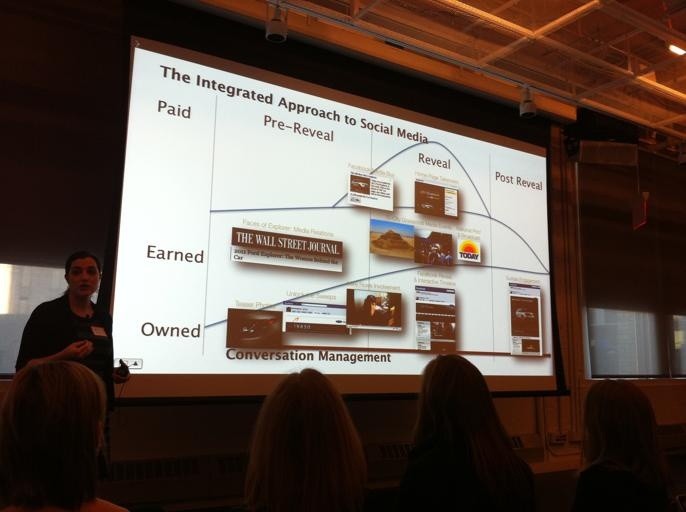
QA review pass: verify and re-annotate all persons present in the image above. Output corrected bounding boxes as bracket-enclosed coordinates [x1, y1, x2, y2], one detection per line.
[571, 379, 676, 510]
[1, 357, 129, 512]
[14, 249, 131, 475]
[244, 368, 376, 511]
[389, 354, 540, 510]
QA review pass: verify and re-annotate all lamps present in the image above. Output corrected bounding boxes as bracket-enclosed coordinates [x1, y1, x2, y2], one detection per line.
[517, 82, 537, 122]
[264, 0, 292, 49]
